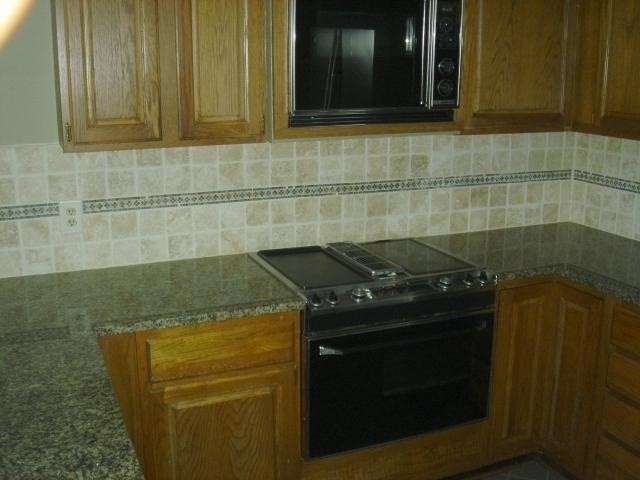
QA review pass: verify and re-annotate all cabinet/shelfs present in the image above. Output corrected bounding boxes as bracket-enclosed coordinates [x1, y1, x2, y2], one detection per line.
[490, 274, 640, 480]
[51, 0, 270, 154]
[97, 309, 303, 480]
[460, 0, 640, 142]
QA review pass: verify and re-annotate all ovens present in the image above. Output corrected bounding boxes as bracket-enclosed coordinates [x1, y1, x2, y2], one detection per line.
[305, 291, 494, 461]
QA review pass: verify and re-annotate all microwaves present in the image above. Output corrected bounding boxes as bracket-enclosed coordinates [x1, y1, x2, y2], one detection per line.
[291, 0, 461, 122]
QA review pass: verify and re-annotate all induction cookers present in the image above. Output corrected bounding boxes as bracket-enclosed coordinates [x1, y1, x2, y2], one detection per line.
[252, 236, 501, 310]
